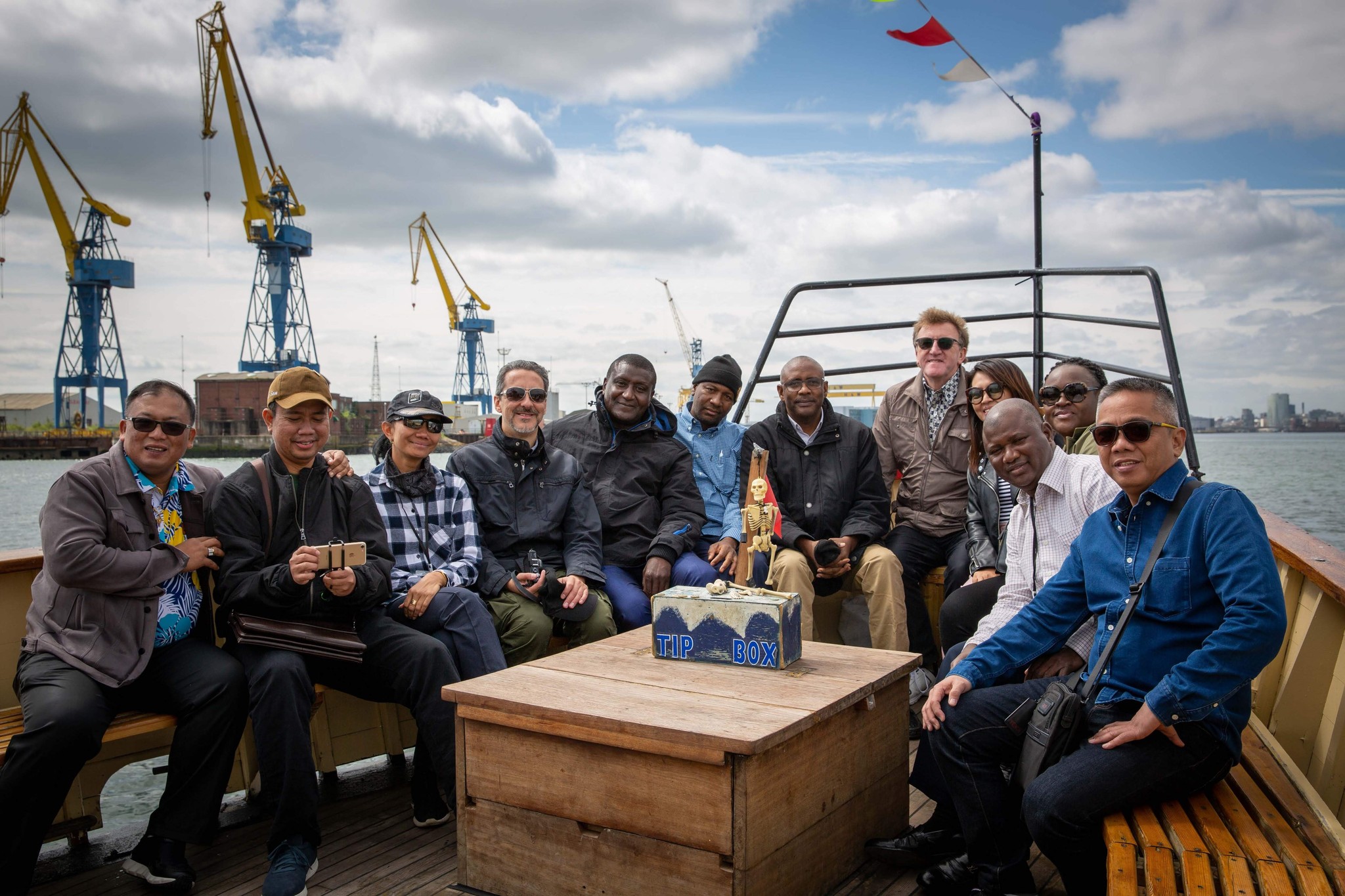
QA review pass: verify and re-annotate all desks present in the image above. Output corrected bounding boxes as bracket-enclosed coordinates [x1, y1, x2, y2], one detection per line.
[441, 624, 921, 896]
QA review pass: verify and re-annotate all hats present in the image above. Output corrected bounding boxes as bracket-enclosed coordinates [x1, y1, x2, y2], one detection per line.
[810, 540, 845, 596]
[387, 390, 453, 425]
[541, 567, 598, 621]
[267, 365, 335, 413]
[692, 353, 743, 403]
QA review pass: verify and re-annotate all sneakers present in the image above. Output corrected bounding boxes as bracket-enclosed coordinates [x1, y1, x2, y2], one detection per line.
[121, 834, 197, 893]
[260, 834, 320, 896]
[908, 667, 936, 705]
[407, 773, 451, 827]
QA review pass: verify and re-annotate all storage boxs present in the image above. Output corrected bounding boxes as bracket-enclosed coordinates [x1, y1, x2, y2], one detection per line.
[651, 583, 803, 669]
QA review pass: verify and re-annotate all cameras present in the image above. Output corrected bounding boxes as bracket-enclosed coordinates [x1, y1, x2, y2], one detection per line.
[524, 549, 543, 586]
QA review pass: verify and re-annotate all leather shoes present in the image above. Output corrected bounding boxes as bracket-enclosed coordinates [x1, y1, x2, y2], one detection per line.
[916, 851, 980, 896]
[863, 823, 967, 857]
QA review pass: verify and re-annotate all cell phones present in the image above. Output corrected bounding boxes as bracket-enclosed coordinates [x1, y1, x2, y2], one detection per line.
[314, 542, 367, 569]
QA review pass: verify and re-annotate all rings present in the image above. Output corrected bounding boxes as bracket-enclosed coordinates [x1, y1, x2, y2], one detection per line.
[208, 546, 214, 556]
[329, 585, 333, 589]
[411, 599, 417, 605]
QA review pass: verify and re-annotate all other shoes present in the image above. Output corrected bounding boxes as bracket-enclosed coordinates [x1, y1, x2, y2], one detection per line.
[909, 706, 922, 738]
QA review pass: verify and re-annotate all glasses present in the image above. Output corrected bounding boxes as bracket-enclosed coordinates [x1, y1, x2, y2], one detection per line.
[1089, 420, 1177, 448]
[1038, 382, 1099, 406]
[390, 415, 443, 434]
[499, 386, 547, 403]
[915, 337, 960, 351]
[783, 377, 826, 392]
[126, 417, 193, 436]
[963, 381, 1005, 405]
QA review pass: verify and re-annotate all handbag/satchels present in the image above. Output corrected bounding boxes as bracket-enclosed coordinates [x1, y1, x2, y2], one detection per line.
[1016, 676, 1082, 794]
[229, 607, 367, 662]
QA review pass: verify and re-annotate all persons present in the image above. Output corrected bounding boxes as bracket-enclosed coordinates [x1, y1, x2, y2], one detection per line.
[960, 359, 1066, 588]
[869, 311, 972, 706]
[939, 357, 1108, 656]
[368, 389, 507, 816]
[670, 354, 773, 591]
[0, 379, 354, 896]
[448, 361, 617, 668]
[210, 367, 460, 896]
[740, 358, 923, 740]
[865, 398, 1124, 863]
[541, 354, 720, 635]
[916, 378, 1287, 896]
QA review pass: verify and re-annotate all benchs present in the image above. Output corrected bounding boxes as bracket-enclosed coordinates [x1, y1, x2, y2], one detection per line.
[0, 642, 446, 848]
[1101, 725, 1345, 896]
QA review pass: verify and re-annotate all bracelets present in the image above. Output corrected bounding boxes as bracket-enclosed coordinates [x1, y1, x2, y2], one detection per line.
[433, 570, 446, 587]
